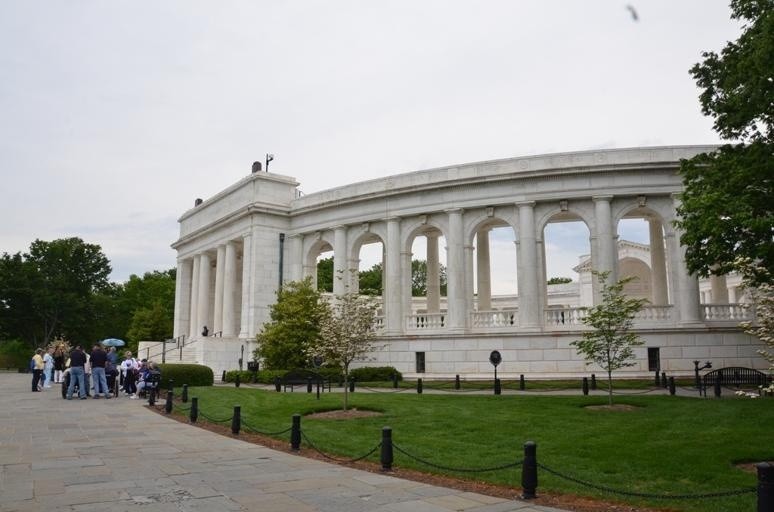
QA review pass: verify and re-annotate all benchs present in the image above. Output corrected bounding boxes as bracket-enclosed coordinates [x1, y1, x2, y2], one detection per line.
[698, 366, 769, 398]
[278, 369, 331, 394]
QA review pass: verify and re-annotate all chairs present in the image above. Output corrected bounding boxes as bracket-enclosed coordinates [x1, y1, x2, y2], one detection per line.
[136, 373, 162, 399]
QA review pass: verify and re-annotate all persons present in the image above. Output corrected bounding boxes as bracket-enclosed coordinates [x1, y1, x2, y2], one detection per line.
[31, 344, 161, 400]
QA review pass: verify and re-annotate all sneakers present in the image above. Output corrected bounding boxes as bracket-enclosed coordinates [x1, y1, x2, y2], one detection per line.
[130, 394, 139, 399]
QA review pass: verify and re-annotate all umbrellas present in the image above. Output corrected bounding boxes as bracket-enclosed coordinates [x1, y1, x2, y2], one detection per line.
[102, 338, 126, 346]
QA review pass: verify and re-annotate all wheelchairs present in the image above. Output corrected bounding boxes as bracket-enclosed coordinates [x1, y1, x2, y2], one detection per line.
[99, 369, 119, 398]
[62, 372, 80, 399]
